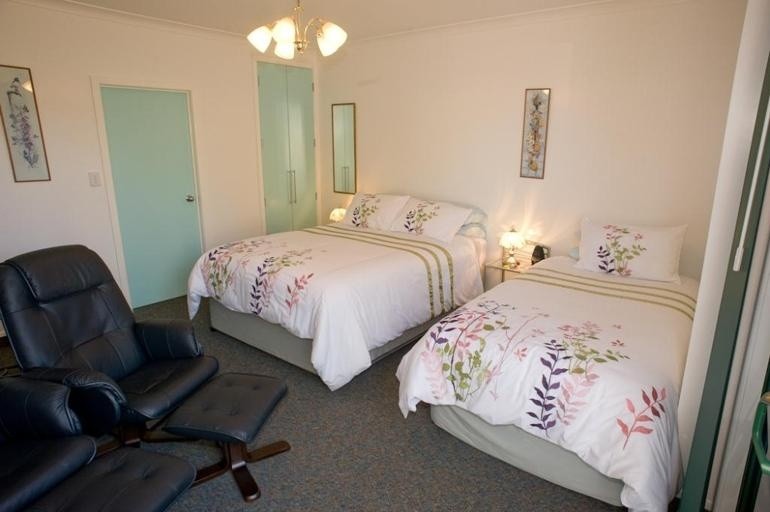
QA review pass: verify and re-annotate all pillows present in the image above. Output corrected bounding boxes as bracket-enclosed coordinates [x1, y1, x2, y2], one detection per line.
[344, 191, 412, 232]
[389, 196, 475, 244]
[571, 213, 690, 286]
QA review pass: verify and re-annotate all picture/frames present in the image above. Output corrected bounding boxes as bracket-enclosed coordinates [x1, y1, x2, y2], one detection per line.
[519, 87, 551, 180]
[0, 65, 53, 181]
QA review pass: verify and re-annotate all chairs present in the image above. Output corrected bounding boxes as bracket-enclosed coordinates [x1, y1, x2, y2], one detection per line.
[1, 241, 222, 461]
[0, 375, 102, 512]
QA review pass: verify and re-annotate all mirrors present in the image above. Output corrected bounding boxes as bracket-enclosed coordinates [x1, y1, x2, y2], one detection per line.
[330, 102, 358, 195]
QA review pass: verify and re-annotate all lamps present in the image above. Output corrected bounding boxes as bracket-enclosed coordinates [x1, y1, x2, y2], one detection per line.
[329, 206, 347, 221]
[245, 2, 350, 61]
[498, 225, 526, 270]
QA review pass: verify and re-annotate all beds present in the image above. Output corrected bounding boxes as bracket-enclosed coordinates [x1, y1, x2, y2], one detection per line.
[393, 253, 701, 511]
[188, 222, 488, 392]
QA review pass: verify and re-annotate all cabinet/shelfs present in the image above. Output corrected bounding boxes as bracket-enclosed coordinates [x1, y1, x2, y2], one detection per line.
[255, 60, 319, 235]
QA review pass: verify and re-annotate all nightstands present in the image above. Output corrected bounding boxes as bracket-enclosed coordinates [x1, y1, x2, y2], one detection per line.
[484, 257, 531, 293]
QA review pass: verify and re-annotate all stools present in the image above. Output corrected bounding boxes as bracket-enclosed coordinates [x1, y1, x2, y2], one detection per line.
[17, 443, 197, 512]
[163, 369, 293, 504]
